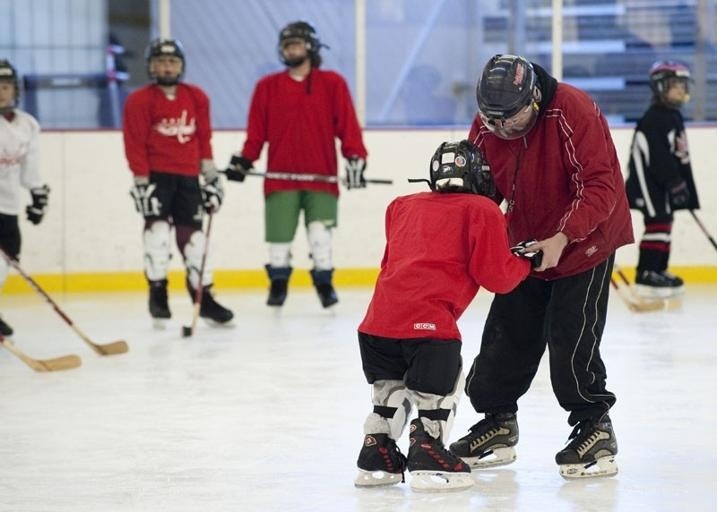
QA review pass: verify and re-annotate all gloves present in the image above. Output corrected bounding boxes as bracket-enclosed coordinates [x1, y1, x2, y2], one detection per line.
[510, 237, 542, 270]
[25, 188, 47, 225]
[344, 156, 367, 188]
[224, 156, 251, 181]
[201, 179, 224, 213]
[129, 184, 162, 220]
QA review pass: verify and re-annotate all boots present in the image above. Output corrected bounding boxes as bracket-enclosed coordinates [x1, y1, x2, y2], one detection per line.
[310, 268, 338, 307]
[357, 411, 619, 474]
[186, 275, 233, 323]
[149, 279, 171, 318]
[266, 264, 292, 306]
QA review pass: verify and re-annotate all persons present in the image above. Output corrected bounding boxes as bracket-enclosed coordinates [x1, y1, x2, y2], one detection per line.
[352, 139, 544, 480]
[624, 62, 700, 288]
[0, 57, 50, 339]
[225, 20, 370, 306]
[123, 40, 234, 325]
[446, 52, 635, 463]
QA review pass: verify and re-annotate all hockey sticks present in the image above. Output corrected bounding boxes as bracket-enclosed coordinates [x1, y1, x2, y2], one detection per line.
[0, 249, 129, 355]
[182, 206, 212, 336]
[609, 264, 684, 312]
[1, 332, 82, 373]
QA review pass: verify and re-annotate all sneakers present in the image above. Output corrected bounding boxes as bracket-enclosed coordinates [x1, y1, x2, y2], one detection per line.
[635, 269, 683, 287]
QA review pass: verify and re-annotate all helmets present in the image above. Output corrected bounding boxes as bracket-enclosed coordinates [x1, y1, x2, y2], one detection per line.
[144, 37, 186, 79]
[279, 21, 320, 54]
[430, 140, 490, 196]
[0, 58, 19, 84]
[648, 60, 696, 110]
[476, 54, 543, 141]
